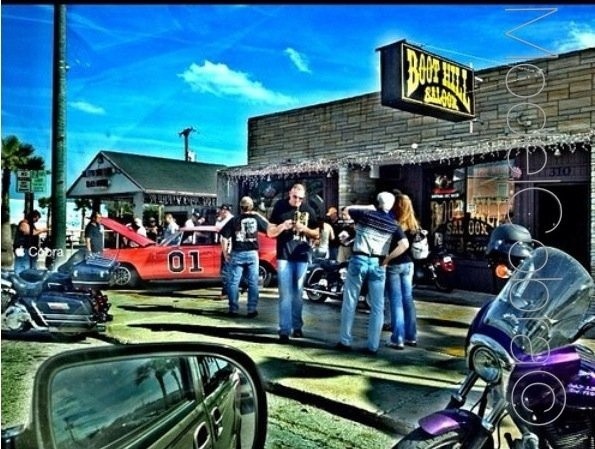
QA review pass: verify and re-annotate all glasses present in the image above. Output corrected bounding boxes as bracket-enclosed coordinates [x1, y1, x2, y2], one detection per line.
[293, 195, 303, 200]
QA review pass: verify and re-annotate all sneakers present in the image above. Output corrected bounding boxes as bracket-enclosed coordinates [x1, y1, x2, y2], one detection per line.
[385, 340, 404, 350]
[248, 311, 257, 318]
[365, 346, 376, 353]
[294, 331, 301, 337]
[383, 322, 391, 330]
[336, 342, 350, 351]
[214, 294, 229, 300]
[279, 335, 288, 342]
[404, 339, 417, 346]
[241, 286, 247, 294]
[229, 310, 239, 315]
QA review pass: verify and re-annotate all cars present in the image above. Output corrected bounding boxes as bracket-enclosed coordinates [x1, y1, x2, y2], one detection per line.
[50, 355, 241, 448]
[235, 374, 253, 402]
[2, 340, 266, 448]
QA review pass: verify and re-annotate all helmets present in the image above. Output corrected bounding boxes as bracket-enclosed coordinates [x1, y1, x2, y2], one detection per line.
[485, 224, 535, 258]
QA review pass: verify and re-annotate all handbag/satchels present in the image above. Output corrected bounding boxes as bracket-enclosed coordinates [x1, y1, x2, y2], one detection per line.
[410, 230, 429, 259]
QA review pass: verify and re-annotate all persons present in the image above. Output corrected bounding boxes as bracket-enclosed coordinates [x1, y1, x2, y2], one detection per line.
[165, 215, 180, 237]
[149, 219, 165, 243]
[338, 206, 356, 261]
[326, 208, 342, 259]
[185, 211, 205, 243]
[267, 184, 321, 343]
[214, 202, 235, 295]
[218, 196, 270, 318]
[336, 191, 410, 357]
[122, 219, 145, 245]
[83, 212, 104, 253]
[13, 211, 51, 272]
[309, 222, 334, 258]
[388, 194, 418, 348]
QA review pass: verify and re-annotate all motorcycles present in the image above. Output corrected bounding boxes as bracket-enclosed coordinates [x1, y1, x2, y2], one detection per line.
[1, 256, 118, 343]
[304, 258, 370, 315]
[393, 245, 594, 448]
[414, 231, 458, 293]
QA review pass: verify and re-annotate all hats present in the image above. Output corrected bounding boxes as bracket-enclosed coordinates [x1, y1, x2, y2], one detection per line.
[193, 212, 200, 218]
[149, 217, 157, 223]
[218, 204, 229, 210]
[240, 196, 253, 206]
[326, 207, 337, 216]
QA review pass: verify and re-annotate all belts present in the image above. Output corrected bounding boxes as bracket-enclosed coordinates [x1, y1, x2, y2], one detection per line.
[353, 252, 385, 257]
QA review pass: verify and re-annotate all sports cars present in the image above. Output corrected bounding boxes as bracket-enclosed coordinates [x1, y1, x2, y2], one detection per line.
[96, 217, 278, 288]
